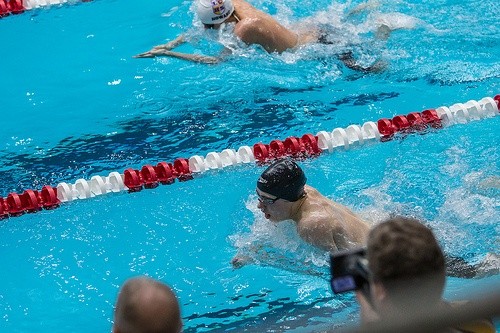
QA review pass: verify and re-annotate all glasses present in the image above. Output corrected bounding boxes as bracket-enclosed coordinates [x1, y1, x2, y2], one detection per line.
[256, 190, 281, 204]
[203, 24, 214, 29]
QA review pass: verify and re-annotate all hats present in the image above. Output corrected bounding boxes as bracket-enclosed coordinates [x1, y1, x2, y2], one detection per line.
[257, 159, 305, 202]
[196, 0, 235, 24]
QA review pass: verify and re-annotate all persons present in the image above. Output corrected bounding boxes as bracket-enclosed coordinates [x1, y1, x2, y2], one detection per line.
[133, 0, 392, 73]
[113, 277, 182, 333]
[230, 159, 495, 333]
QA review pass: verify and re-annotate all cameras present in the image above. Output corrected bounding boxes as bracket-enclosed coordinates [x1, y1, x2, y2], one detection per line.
[329, 249, 367, 294]
